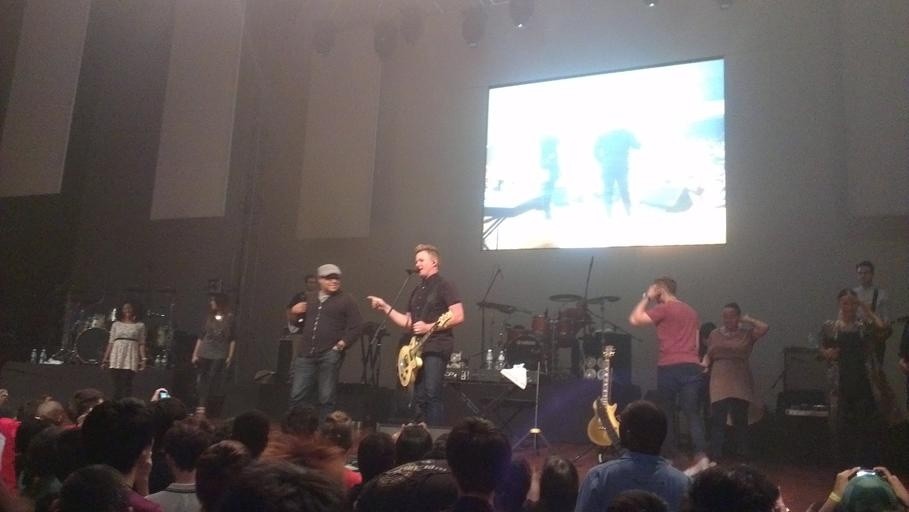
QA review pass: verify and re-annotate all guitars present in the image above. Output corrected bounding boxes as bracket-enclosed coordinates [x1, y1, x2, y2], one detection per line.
[587, 345, 620, 447]
[398, 312, 453, 387]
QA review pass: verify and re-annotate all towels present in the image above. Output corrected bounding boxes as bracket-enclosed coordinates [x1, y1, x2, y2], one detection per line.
[500, 362, 528, 391]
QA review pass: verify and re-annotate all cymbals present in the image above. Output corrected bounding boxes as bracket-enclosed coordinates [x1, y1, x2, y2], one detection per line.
[587, 296, 620, 305]
[549, 294, 583, 303]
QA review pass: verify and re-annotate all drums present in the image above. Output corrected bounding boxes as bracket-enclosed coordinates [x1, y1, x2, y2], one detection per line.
[506, 335, 543, 371]
[532, 314, 545, 332]
[76, 326, 110, 363]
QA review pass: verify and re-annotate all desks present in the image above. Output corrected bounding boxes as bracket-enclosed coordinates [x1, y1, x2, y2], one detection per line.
[480, 396, 535, 437]
[444, 369, 544, 420]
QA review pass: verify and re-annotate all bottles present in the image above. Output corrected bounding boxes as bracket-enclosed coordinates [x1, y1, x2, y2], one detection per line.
[155, 355, 161, 367]
[486, 350, 492, 369]
[30, 349, 37, 364]
[40, 349, 46, 364]
[161, 355, 167, 366]
[498, 351, 504, 369]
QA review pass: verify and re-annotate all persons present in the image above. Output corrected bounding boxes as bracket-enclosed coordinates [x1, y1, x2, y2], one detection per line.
[0, 387, 355, 512]
[575, 400, 691, 512]
[699, 321, 733, 426]
[285, 263, 364, 415]
[492, 457, 535, 512]
[392, 422, 434, 466]
[837, 260, 897, 411]
[684, 464, 788, 512]
[629, 277, 709, 462]
[355, 433, 457, 512]
[190, 292, 237, 415]
[347, 433, 395, 501]
[820, 286, 887, 381]
[446, 415, 512, 512]
[285, 273, 317, 308]
[827, 335, 897, 438]
[538, 454, 580, 512]
[367, 243, 465, 428]
[99, 300, 148, 398]
[700, 301, 769, 459]
[818, 464, 909, 512]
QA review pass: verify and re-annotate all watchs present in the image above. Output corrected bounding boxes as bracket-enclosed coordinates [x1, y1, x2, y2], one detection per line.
[642, 292, 651, 302]
[335, 341, 344, 351]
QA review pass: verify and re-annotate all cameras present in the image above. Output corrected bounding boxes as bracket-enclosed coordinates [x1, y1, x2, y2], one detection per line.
[855, 469, 880, 478]
[158, 390, 172, 399]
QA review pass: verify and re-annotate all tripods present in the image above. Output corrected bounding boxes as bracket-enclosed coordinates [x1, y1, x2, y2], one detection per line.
[511, 356, 555, 458]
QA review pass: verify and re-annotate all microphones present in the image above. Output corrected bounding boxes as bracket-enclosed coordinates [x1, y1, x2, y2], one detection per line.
[405, 267, 420, 274]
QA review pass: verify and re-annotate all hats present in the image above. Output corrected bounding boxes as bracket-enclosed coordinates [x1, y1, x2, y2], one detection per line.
[842, 475, 897, 512]
[318, 264, 341, 280]
[37, 396, 65, 422]
[355, 458, 457, 512]
